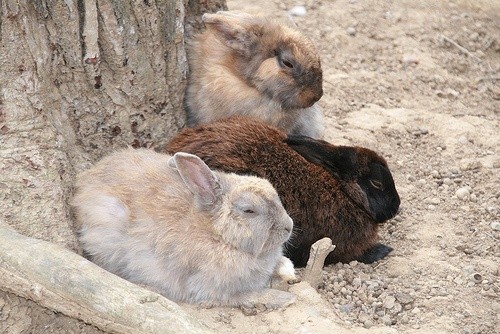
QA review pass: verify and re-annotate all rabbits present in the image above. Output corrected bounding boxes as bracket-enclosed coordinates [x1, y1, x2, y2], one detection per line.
[70, 147, 296, 305]
[184, 11, 325, 138]
[163, 118, 402, 267]
